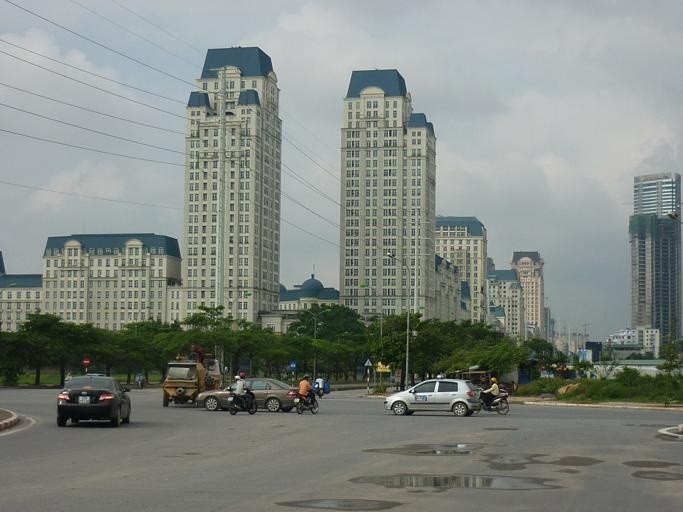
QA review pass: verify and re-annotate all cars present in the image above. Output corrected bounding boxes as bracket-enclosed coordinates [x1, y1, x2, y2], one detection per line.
[196, 377, 300, 412]
[383, 375, 479, 417]
[55, 374, 132, 428]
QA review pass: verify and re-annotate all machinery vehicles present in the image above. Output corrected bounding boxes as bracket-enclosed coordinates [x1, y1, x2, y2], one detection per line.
[160, 343, 222, 407]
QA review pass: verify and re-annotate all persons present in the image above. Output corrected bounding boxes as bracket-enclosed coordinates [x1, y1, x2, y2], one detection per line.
[299, 374, 315, 408]
[235, 371, 250, 409]
[235, 374, 241, 382]
[480, 377, 499, 408]
[65, 373, 72, 384]
[316, 376, 324, 395]
[136, 373, 143, 389]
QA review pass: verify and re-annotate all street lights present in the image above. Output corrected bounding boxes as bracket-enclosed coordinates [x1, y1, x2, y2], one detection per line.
[386, 252, 410, 390]
[304, 309, 333, 380]
[411, 330, 418, 386]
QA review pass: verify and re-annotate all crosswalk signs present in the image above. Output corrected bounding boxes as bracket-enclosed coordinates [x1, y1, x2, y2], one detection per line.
[289, 360, 296, 369]
[364, 358, 373, 367]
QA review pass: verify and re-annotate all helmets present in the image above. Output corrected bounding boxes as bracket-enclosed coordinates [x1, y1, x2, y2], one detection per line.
[491, 378, 497, 383]
[239, 372, 246, 377]
[303, 374, 309, 378]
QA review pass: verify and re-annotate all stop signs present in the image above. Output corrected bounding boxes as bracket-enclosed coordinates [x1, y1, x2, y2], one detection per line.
[82, 356, 90, 367]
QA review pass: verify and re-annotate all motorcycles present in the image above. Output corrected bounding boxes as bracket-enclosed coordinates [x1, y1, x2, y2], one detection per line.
[472, 386, 509, 416]
[136, 379, 145, 389]
[227, 387, 258, 415]
[290, 390, 319, 414]
